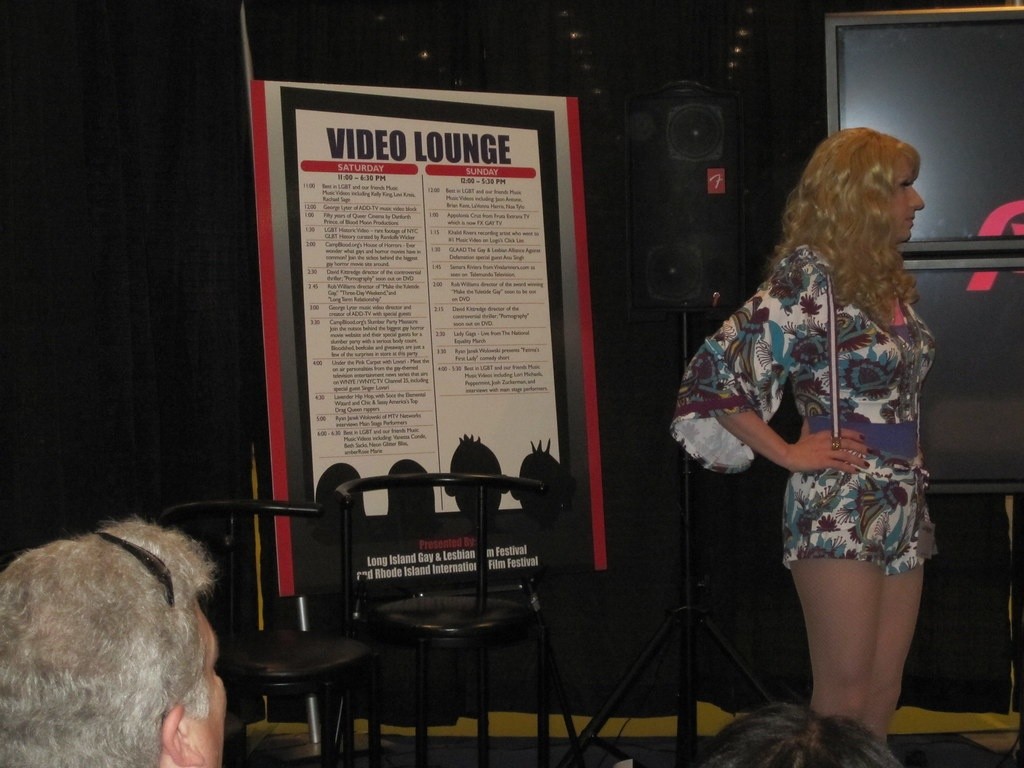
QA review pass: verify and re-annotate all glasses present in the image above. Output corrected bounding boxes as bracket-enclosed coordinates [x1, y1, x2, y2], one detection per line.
[96, 532, 174, 606]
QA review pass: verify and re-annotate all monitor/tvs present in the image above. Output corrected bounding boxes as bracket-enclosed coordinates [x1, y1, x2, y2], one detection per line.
[824, 4, 1024, 252]
[902, 257, 1024, 493]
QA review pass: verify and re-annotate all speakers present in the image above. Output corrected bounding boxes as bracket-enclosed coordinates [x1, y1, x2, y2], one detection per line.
[624, 81, 747, 312]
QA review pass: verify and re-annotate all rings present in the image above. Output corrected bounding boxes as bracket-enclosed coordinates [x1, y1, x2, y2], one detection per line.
[832, 437, 842, 450]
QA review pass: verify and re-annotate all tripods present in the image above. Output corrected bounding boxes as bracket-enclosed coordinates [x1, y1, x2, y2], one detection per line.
[557, 309, 777, 768]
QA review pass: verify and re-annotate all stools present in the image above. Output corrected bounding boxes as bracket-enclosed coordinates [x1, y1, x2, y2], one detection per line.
[333, 473, 551, 768]
[149, 501, 382, 768]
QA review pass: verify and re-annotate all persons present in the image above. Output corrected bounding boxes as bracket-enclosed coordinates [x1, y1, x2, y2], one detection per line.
[671, 126, 936, 743]
[0, 517, 226, 768]
[696, 703, 902, 768]
